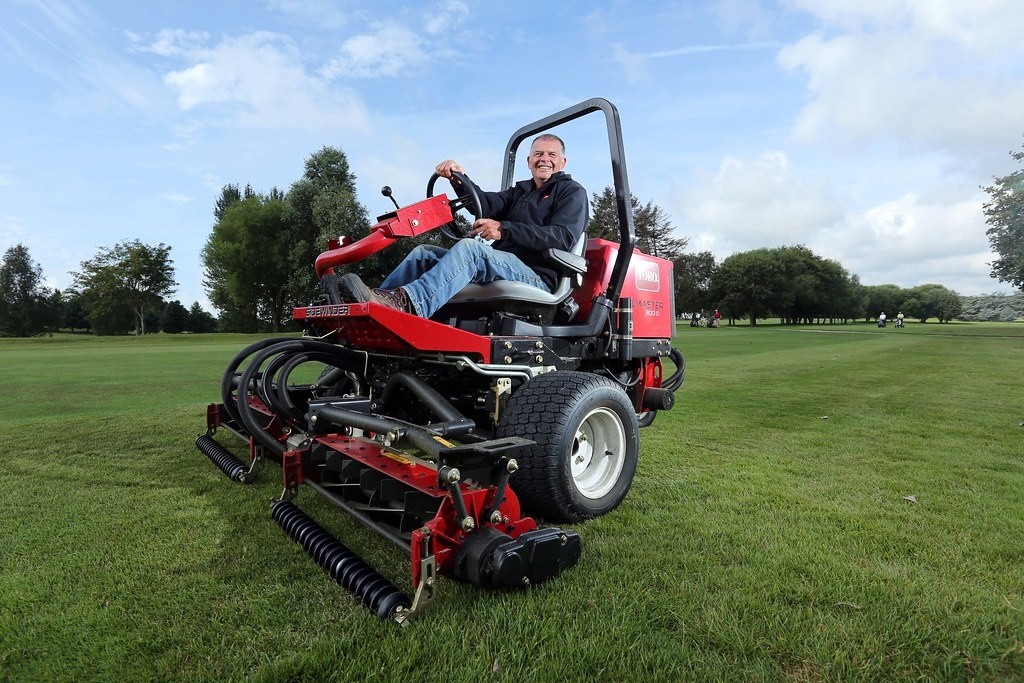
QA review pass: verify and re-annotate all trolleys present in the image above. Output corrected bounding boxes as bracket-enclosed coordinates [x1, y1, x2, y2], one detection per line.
[894, 318, 905, 328]
[877, 319, 886, 327]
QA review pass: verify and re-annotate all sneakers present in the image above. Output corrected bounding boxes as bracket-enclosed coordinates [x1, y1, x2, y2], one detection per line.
[322, 272, 410, 317]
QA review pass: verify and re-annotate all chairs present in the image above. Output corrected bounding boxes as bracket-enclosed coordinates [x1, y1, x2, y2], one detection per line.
[444, 228, 590, 325]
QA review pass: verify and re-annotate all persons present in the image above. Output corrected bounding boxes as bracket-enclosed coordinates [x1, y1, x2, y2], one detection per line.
[314, 132, 590, 322]
[689, 307, 723, 328]
[878, 311, 905, 329]
